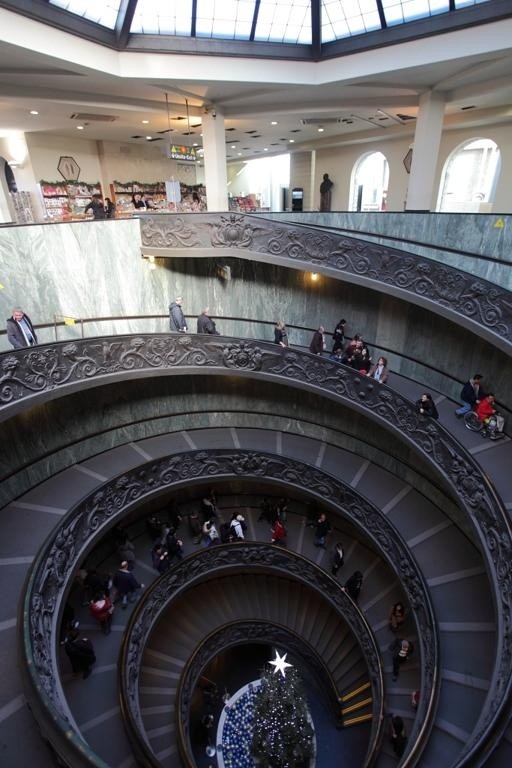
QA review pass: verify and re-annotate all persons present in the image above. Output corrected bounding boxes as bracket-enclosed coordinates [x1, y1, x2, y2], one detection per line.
[274, 315, 506, 442]
[320, 172, 332, 191]
[168, 294, 188, 332]
[83, 194, 105, 220]
[263, 493, 421, 757]
[59, 481, 248, 680]
[197, 304, 221, 334]
[190, 657, 258, 748]
[131, 192, 144, 207]
[6, 306, 38, 349]
[104, 197, 115, 217]
[188, 192, 200, 210]
